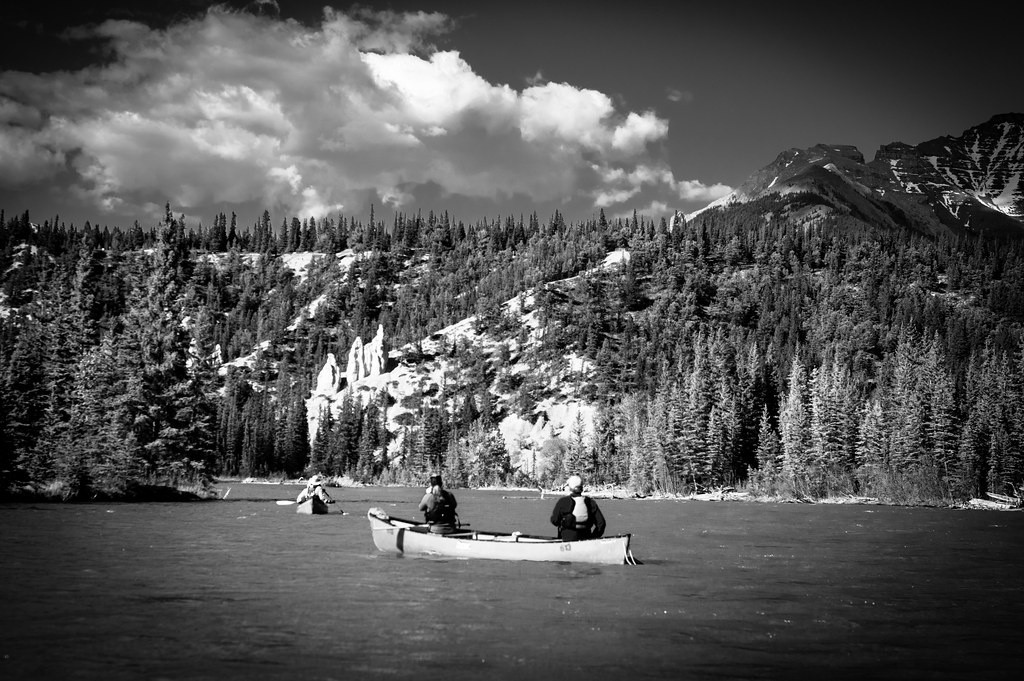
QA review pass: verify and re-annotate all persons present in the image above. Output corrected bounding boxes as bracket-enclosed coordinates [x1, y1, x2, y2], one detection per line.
[550, 476, 607, 541]
[297, 486, 313, 504]
[419, 475, 457, 534]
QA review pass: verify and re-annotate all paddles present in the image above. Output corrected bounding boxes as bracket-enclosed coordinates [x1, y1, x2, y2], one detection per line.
[275, 498, 317, 507]
[371, 522, 472, 532]
[322, 486, 345, 513]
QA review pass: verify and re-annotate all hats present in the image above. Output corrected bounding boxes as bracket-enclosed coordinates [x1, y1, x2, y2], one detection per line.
[563, 476, 583, 491]
[311, 474, 323, 484]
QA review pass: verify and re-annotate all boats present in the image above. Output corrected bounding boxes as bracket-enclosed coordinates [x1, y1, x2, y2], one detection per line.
[296, 494, 328, 514]
[367, 507, 631, 565]
